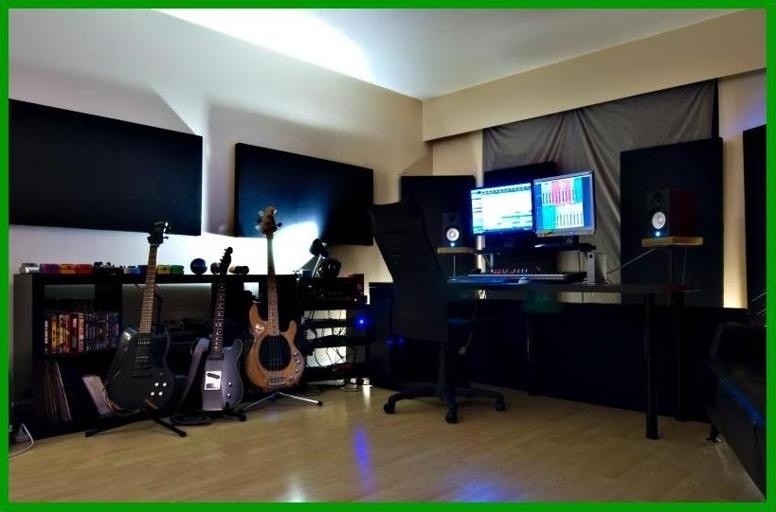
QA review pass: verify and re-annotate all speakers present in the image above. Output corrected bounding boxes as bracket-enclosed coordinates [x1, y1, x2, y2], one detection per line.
[437, 213, 474, 254]
[640, 188, 705, 247]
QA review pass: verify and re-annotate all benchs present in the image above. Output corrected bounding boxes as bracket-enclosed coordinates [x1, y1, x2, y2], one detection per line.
[705, 321, 766, 499]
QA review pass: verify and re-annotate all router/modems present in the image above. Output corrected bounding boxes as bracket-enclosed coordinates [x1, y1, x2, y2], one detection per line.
[587, 251, 607, 285]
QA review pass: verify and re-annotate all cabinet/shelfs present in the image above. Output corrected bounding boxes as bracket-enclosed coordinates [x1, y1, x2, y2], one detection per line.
[13, 273, 370, 438]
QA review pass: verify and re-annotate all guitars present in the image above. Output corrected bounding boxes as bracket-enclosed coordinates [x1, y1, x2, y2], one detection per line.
[177, 247, 244, 414]
[105, 220, 176, 408]
[243, 205, 305, 393]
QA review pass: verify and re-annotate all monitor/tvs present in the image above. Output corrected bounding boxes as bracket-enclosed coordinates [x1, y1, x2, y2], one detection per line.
[469, 180, 536, 254]
[533, 170, 596, 250]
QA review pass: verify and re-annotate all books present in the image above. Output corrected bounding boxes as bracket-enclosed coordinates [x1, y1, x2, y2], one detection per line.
[44, 360, 71, 422]
[641, 235, 704, 247]
[42, 309, 120, 355]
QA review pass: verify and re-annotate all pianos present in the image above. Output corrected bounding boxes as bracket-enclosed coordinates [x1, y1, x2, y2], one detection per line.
[447, 267, 587, 288]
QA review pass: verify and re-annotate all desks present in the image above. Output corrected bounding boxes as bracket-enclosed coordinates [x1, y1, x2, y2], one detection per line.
[445, 281, 676, 437]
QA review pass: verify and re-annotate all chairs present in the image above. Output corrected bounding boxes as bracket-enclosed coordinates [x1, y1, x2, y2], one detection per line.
[366, 202, 509, 423]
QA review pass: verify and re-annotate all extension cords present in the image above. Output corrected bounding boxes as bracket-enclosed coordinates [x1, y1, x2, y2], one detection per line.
[13, 432, 29, 442]
